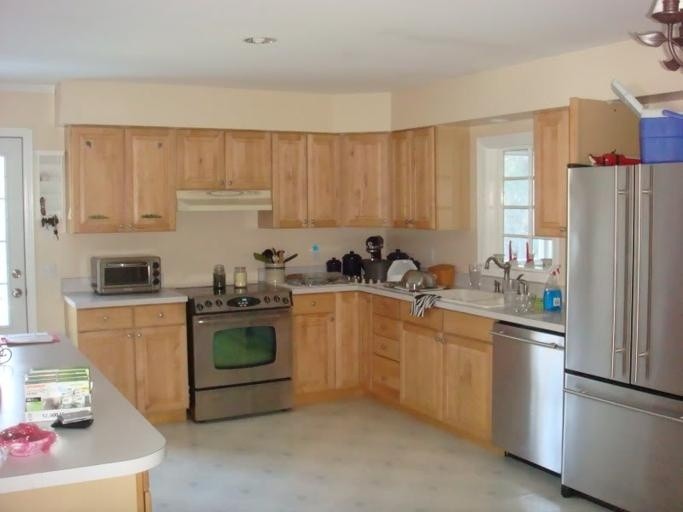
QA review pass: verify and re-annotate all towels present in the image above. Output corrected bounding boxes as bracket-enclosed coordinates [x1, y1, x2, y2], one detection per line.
[409, 294, 441, 319]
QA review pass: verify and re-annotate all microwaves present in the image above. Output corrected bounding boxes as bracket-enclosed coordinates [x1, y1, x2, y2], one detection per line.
[90, 256, 162, 295]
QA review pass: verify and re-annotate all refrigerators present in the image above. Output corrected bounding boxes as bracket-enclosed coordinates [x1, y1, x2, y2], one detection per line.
[561, 162, 683, 512]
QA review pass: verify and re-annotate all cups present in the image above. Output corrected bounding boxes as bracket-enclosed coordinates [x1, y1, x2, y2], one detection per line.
[264, 264, 285, 284]
[494, 254, 504, 263]
[541, 258, 552, 271]
[469, 263, 482, 290]
[501, 279, 519, 315]
[515, 294, 535, 317]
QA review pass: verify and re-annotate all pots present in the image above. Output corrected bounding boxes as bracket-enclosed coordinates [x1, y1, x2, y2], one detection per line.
[325, 256, 343, 273]
[342, 251, 361, 276]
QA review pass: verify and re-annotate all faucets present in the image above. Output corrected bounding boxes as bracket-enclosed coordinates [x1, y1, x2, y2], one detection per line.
[483, 256, 529, 300]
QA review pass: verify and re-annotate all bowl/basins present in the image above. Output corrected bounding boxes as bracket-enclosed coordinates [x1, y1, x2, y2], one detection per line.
[399, 270, 437, 292]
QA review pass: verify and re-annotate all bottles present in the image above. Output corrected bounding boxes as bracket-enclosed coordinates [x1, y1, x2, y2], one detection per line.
[213, 264, 226, 294]
[234, 267, 248, 294]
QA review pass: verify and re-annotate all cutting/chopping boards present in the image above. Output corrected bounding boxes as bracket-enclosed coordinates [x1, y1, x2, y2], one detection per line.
[285, 272, 338, 287]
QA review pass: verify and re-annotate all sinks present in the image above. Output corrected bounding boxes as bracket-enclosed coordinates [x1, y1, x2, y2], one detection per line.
[420, 287, 508, 310]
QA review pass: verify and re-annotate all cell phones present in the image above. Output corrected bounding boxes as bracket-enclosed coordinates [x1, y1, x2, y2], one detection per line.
[58, 409, 92, 423]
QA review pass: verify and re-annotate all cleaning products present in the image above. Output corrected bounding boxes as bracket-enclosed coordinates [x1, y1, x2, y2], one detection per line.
[542, 269, 563, 312]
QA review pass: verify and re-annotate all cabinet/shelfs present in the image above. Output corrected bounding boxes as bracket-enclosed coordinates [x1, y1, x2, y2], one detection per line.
[366, 281, 404, 404]
[64, 288, 191, 426]
[175, 128, 271, 206]
[342, 133, 389, 229]
[0, 334, 166, 512]
[401, 287, 501, 452]
[290, 278, 363, 404]
[63, 123, 176, 234]
[389, 125, 470, 232]
[257, 132, 342, 228]
[535, 99, 643, 238]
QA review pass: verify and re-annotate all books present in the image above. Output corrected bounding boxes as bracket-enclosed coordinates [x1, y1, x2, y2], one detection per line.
[23, 367, 91, 427]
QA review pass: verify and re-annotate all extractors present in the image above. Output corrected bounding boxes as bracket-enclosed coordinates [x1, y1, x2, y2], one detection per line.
[176, 190, 272, 213]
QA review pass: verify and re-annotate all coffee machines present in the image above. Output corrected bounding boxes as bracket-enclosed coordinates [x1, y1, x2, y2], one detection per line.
[361, 236, 393, 284]
